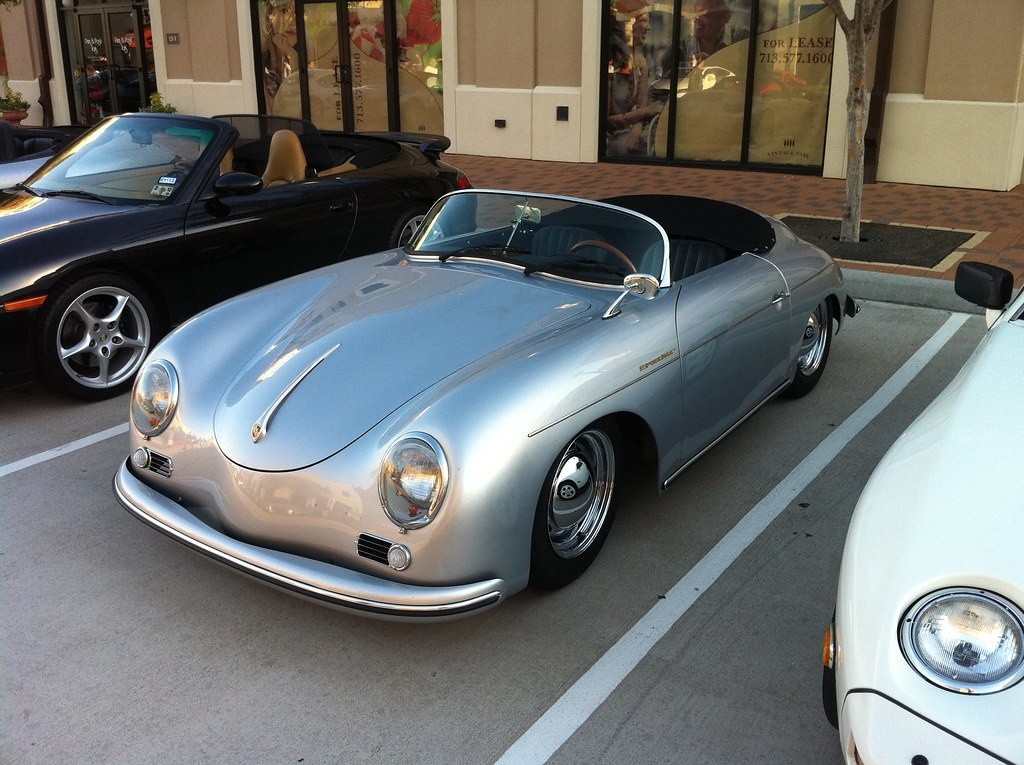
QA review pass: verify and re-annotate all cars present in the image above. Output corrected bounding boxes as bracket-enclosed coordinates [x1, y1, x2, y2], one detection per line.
[821, 261, 1024, 765]
[48, 10, 831, 176]
[112, 182, 864, 626]
[0, 103, 483, 408]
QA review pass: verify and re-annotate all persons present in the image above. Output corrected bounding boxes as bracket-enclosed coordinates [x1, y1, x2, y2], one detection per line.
[608, 0, 664, 156]
[661, 0, 732, 80]
[260, 0, 301, 115]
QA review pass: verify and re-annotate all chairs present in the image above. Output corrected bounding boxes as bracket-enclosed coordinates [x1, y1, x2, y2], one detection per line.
[637, 238, 729, 286]
[258, 129, 311, 191]
[189, 128, 237, 178]
[507, 224, 609, 273]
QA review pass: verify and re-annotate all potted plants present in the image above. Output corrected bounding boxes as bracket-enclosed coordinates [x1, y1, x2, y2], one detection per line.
[0, 87, 32, 128]
